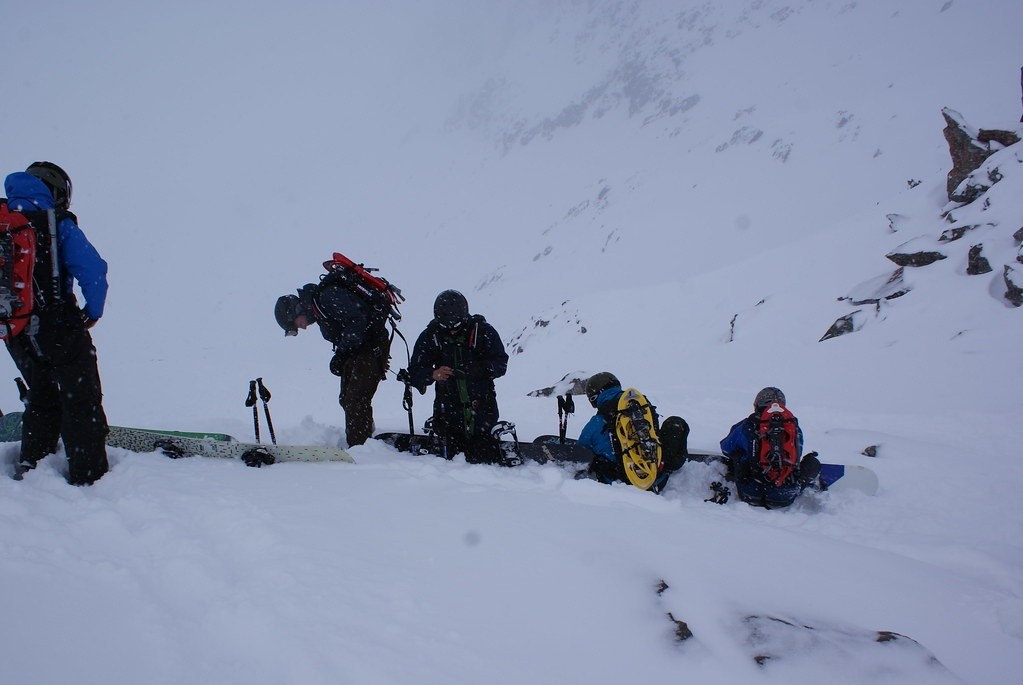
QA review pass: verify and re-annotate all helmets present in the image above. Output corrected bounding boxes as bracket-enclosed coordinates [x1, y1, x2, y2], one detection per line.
[753, 387, 786, 409]
[274, 295, 299, 336]
[586, 372, 621, 407]
[26, 161, 72, 209]
[434, 290, 468, 331]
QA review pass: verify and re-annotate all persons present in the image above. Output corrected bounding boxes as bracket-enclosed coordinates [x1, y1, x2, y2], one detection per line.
[407, 288, 509, 464]
[274, 266, 391, 449]
[720, 387, 821, 511]
[576, 371, 691, 495]
[0, 159, 111, 488]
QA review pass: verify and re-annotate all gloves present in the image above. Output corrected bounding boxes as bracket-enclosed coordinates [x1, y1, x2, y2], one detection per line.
[330, 354, 342, 377]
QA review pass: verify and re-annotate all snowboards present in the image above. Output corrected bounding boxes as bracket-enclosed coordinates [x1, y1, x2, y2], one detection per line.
[371, 432, 880, 501]
[0, 410, 357, 470]
